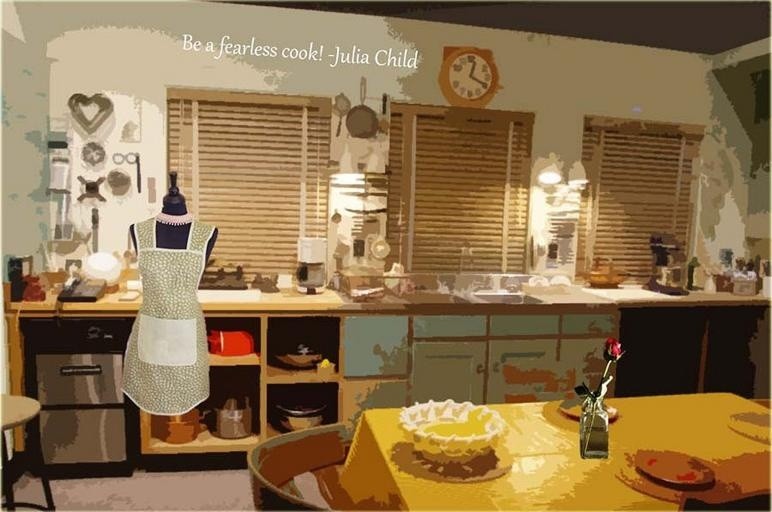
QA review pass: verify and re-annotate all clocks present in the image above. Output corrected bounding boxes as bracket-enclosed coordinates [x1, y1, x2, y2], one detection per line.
[438, 46, 498, 110]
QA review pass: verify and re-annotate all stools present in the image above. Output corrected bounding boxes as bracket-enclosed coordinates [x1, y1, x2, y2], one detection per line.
[1, 394, 56, 512]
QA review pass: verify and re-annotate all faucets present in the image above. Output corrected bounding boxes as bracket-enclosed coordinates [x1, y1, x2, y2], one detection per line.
[459, 240, 475, 276]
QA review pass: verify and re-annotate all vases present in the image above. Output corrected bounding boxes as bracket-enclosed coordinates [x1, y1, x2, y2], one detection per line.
[578, 396, 610, 459]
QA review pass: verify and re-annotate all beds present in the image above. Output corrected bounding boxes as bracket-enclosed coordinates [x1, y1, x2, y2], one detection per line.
[364, 391, 771, 511]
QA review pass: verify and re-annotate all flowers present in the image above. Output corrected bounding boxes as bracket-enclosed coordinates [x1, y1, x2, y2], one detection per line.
[574, 337, 626, 453]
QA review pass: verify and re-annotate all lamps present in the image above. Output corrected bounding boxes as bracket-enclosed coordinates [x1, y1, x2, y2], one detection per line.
[538, 160, 590, 187]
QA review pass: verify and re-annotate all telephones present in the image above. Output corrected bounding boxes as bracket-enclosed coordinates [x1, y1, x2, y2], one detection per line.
[56, 275, 107, 302]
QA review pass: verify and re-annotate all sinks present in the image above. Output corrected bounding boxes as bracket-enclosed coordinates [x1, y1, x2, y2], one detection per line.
[454, 290, 543, 304]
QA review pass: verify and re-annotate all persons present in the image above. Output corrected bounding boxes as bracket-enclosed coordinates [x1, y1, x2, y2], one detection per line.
[121, 193, 218, 416]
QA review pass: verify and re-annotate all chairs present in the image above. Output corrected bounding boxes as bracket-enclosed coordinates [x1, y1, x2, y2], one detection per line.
[247, 421, 347, 511]
